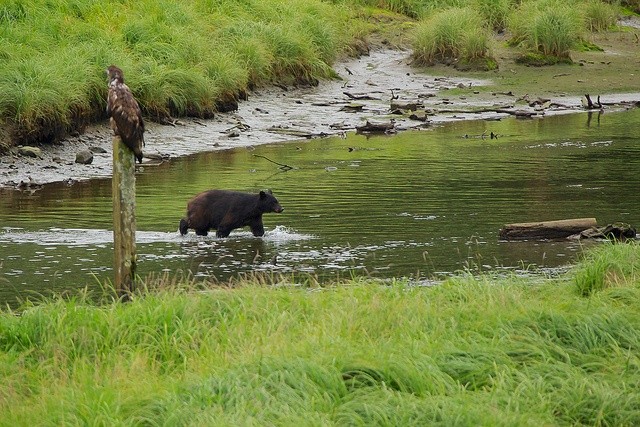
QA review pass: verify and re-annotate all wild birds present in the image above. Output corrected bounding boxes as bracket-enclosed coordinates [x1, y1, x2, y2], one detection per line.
[106, 63, 147, 166]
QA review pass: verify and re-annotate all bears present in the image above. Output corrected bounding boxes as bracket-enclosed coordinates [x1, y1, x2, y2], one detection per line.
[179, 188, 284, 239]
